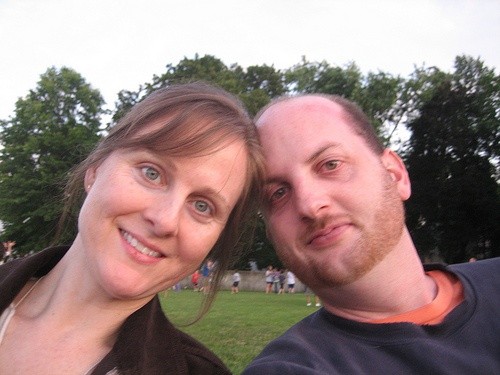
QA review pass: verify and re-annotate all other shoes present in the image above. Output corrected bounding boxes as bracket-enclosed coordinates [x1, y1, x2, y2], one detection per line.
[315, 303, 320, 306]
[306, 303, 311, 306]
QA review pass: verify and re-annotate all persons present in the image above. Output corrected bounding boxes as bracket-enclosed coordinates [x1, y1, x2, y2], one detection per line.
[230, 271, 240, 294]
[0, 80, 268, 374]
[265, 265, 324, 307]
[184, 260, 216, 294]
[242, 92, 500, 375]
